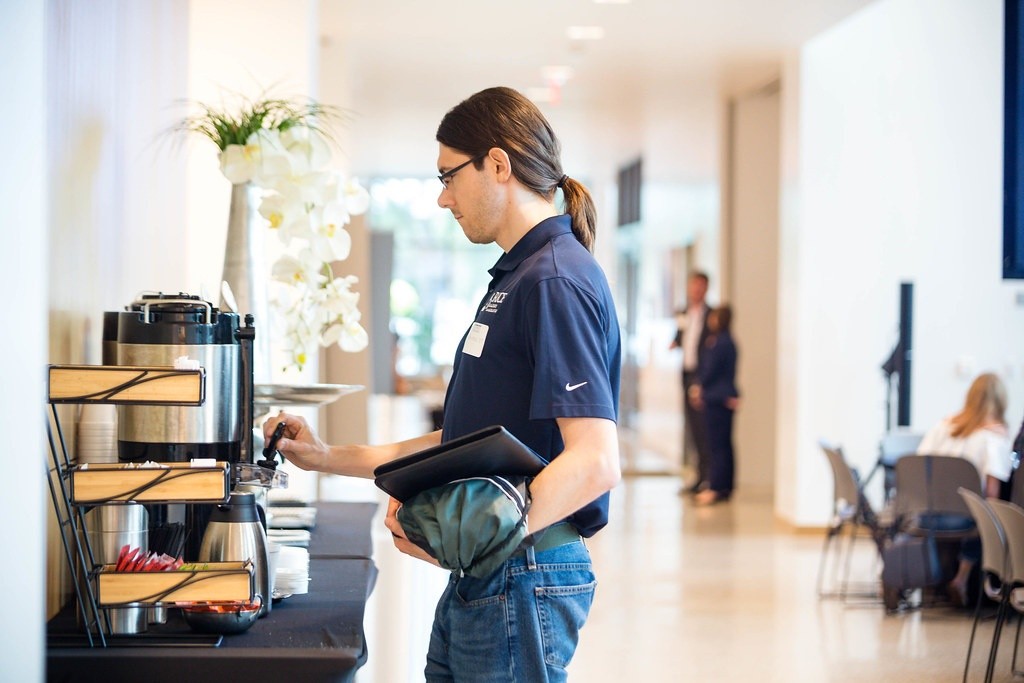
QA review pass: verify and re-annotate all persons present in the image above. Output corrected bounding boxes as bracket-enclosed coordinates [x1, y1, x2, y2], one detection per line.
[264, 86, 622, 683]
[668, 270, 740, 505]
[915, 370, 1014, 612]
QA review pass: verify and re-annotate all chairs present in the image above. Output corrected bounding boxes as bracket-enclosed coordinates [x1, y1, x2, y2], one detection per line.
[814, 432, 1024, 683]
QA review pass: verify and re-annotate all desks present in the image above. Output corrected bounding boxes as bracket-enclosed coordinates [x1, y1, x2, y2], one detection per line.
[46, 501, 380, 683]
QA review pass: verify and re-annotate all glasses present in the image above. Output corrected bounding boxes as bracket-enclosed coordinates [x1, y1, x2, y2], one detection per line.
[436, 150, 490, 190]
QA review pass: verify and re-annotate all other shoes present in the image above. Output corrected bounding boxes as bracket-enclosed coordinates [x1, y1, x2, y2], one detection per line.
[690, 489, 732, 508]
[678, 479, 704, 495]
[944, 579, 969, 610]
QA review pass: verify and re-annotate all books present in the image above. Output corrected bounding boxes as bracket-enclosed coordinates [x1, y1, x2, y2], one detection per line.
[372, 423, 550, 505]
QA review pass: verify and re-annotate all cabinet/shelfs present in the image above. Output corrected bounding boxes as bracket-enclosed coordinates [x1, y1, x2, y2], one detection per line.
[46, 363, 257, 647]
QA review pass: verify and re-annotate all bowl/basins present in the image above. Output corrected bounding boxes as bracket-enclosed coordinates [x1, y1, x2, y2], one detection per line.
[182, 593, 263, 635]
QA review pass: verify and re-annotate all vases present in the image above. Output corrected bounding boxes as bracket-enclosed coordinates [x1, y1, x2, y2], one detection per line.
[217, 181, 264, 384]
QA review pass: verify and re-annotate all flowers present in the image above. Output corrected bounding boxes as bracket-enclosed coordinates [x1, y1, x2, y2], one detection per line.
[159, 83, 372, 373]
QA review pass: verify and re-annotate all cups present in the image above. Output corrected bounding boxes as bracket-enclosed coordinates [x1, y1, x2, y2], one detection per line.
[268, 544, 281, 592]
[78, 403, 118, 463]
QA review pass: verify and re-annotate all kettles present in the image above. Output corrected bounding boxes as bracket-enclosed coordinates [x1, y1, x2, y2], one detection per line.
[200, 493, 272, 615]
